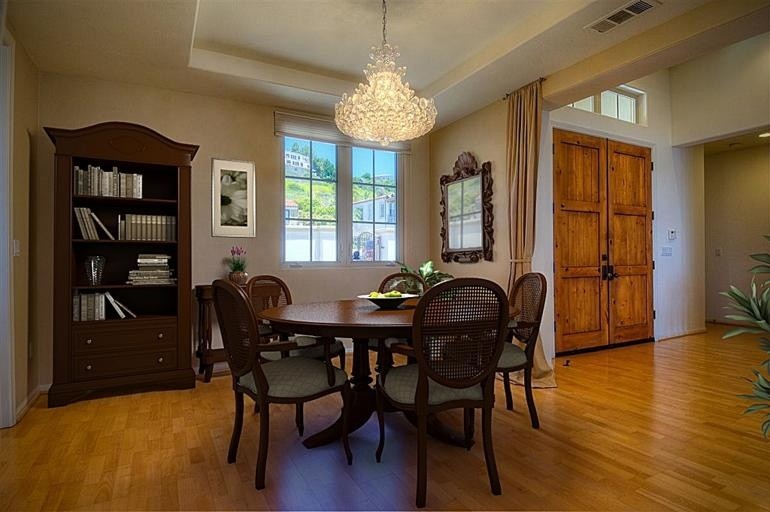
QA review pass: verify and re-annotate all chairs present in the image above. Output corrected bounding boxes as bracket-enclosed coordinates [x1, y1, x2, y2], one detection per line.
[370, 272, 427, 364]
[374, 275, 512, 509]
[206, 277, 355, 491]
[444, 269, 551, 430]
[245, 272, 349, 418]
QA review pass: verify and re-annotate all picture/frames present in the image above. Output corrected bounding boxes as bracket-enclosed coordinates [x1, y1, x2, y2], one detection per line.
[209, 156, 259, 240]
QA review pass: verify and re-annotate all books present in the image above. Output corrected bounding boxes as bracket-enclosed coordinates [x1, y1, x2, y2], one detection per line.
[70, 163, 178, 324]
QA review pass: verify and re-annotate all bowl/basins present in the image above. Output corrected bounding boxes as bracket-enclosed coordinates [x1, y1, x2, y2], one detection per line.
[357, 293, 419, 309]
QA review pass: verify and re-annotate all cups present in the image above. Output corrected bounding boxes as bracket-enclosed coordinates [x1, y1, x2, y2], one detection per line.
[83, 255, 108, 286]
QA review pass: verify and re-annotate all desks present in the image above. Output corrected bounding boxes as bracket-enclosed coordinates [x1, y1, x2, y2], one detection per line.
[253, 293, 521, 450]
[195, 282, 282, 384]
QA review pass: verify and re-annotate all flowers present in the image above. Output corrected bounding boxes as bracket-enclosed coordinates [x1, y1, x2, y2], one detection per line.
[225, 243, 248, 271]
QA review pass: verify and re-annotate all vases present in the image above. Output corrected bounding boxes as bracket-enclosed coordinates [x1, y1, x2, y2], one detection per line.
[230, 271, 248, 286]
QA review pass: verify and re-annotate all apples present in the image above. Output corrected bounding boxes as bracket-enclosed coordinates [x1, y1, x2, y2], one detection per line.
[369, 290, 402, 298]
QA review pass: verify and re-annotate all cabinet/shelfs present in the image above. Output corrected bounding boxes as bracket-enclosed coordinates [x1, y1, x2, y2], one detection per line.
[39, 119, 205, 409]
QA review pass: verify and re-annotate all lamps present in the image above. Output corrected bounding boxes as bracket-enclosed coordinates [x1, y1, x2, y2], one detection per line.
[333, 1, 440, 151]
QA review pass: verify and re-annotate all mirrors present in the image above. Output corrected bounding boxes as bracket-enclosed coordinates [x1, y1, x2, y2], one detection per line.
[437, 150, 495, 267]
